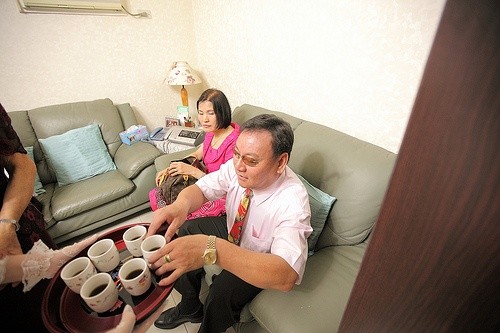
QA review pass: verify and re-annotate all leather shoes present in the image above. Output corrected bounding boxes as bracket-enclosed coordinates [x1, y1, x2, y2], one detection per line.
[154, 303, 204, 329]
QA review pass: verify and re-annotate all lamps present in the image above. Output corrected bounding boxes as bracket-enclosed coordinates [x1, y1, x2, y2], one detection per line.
[163, 61, 203, 105]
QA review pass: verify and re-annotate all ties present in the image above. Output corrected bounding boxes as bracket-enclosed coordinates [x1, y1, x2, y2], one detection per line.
[227, 188, 253, 247]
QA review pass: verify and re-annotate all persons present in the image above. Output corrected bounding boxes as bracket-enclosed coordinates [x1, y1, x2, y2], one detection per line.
[0, 234, 168, 333]
[0, 104, 54, 288]
[146, 114, 313, 333]
[148, 89, 241, 223]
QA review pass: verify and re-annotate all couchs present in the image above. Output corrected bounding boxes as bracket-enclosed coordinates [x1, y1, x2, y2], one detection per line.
[0, 98, 162, 246]
[153, 103, 398, 333]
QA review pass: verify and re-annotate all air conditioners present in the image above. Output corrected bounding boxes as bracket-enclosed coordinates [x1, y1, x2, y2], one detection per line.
[18, 0, 125, 16]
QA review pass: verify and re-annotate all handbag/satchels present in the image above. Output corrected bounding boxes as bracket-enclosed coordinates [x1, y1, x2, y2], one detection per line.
[156, 156, 209, 205]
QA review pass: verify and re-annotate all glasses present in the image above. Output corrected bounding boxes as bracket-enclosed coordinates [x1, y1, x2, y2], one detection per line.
[232, 145, 283, 168]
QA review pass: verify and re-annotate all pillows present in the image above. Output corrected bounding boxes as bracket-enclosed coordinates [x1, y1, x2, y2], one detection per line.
[295, 172, 337, 257]
[24, 146, 46, 197]
[37, 124, 116, 188]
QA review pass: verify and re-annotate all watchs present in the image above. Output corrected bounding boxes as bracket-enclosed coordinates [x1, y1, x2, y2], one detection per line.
[201, 236, 217, 265]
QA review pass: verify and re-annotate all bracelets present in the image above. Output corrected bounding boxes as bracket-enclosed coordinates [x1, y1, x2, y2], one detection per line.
[0, 219, 20, 231]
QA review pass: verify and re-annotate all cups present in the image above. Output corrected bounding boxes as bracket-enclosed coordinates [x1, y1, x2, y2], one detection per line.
[140, 234, 166, 270]
[87, 239, 121, 273]
[118, 258, 152, 296]
[122, 226, 147, 258]
[60, 257, 98, 294]
[80, 273, 118, 313]
[185, 121, 195, 127]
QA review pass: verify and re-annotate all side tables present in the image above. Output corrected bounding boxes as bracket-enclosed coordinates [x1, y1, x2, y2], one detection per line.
[151, 122, 204, 154]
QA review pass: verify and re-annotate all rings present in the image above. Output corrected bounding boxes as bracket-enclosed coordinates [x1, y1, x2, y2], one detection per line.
[165, 255, 171, 262]
[175, 169, 177, 172]
